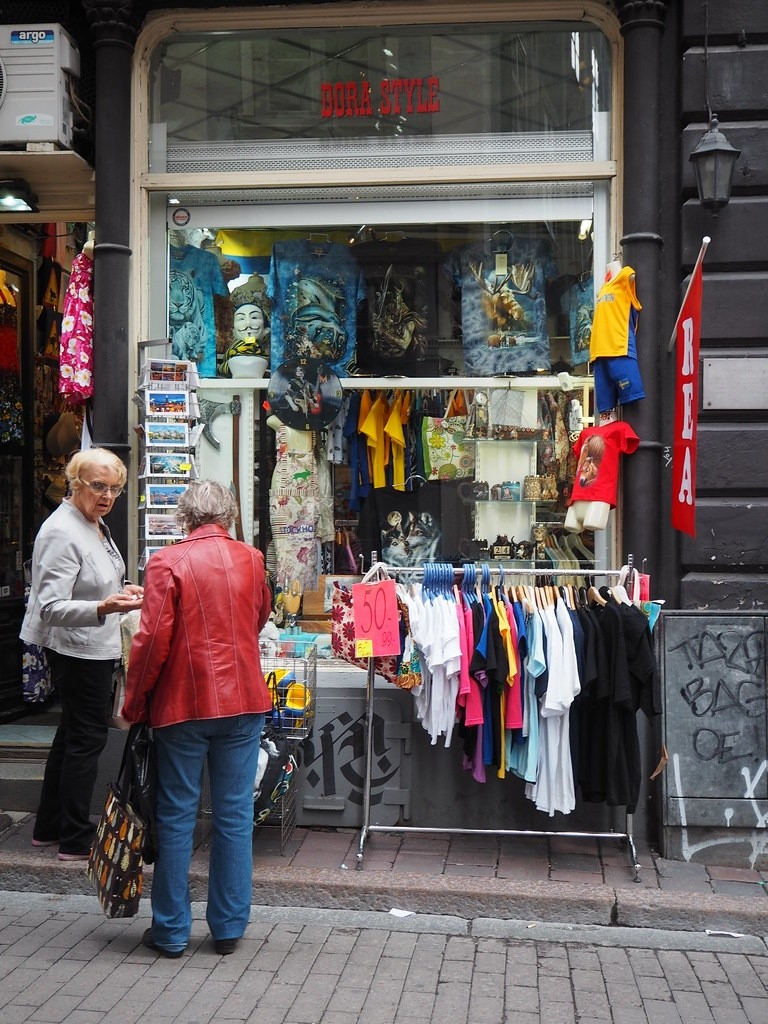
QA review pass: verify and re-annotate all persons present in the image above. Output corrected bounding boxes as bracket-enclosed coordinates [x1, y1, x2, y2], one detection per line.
[23, 558, 54, 705]
[589, 260, 647, 413]
[563, 412, 640, 533]
[18, 447, 145, 861]
[224, 276, 271, 356]
[266, 415, 335, 593]
[121, 477, 273, 958]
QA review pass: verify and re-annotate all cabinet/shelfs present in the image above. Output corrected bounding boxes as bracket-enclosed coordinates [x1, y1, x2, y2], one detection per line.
[460, 436, 600, 564]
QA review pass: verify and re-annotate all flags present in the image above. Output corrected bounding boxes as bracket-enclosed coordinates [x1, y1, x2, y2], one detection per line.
[670, 256, 702, 539]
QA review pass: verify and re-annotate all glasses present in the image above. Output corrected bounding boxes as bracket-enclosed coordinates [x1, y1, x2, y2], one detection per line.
[80, 477, 123, 498]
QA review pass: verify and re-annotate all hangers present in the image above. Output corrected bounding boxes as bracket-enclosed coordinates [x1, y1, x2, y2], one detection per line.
[548, 569, 554, 605]
[552, 568, 633, 611]
[486, 564, 490, 593]
[460, 564, 471, 609]
[539, 568, 548, 609]
[502, 566, 509, 599]
[336, 519, 350, 546]
[386, 388, 439, 416]
[473, 564, 480, 603]
[543, 568, 552, 606]
[469, 564, 475, 601]
[465, 564, 473, 604]
[522, 568, 531, 600]
[480, 564, 485, 599]
[483, 564, 488, 595]
[394, 563, 455, 606]
[495, 564, 509, 604]
[508, 568, 517, 602]
[534, 568, 542, 610]
[515, 567, 534, 613]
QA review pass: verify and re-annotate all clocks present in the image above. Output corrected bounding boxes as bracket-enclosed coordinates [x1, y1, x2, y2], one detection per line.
[267, 356, 343, 431]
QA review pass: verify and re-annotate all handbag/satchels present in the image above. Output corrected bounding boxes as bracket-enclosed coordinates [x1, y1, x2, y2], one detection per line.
[84, 721, 153, 918]
[331, 562, 405, 684]
[253, 672, 298, 829]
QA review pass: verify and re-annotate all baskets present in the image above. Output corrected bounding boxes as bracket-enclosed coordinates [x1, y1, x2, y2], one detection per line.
[258, 640, 318, 739]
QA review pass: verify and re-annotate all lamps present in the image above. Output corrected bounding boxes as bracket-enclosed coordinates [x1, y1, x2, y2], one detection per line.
[0, 176, 40, 213]
[688, 113, 742, 219]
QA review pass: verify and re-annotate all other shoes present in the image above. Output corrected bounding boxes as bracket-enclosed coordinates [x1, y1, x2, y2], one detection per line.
[212, 938, 236, 954]
[30, 834, 58, 847]
[142, 928, 186, 958]
[58, 842, 90, 860]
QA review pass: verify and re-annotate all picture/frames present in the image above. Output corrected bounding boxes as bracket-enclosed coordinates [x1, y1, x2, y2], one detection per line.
[145, 421, 190, 447]
[146, 483, 190, 509]
[145, 358, 190, 384]
[145, 546, 166, 562]
[144, 514, 185, 540]
[145, 390, 189, 416]
[145, 452, 190, 478]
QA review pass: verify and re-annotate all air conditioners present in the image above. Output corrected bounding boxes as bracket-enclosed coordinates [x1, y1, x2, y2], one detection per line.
[0, 23, 81, 150]
